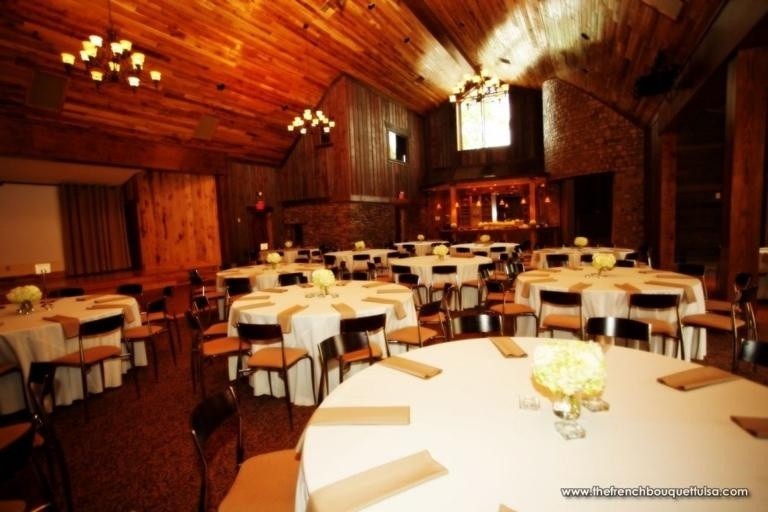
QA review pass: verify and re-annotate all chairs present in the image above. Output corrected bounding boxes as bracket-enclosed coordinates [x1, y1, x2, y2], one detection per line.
[1, 225, 768, 512]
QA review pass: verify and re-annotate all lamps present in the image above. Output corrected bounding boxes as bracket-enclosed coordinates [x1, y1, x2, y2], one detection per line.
[448, 63, 510, 113]
[59, 0, 162, 96]
[285, 23, 339, 136]
